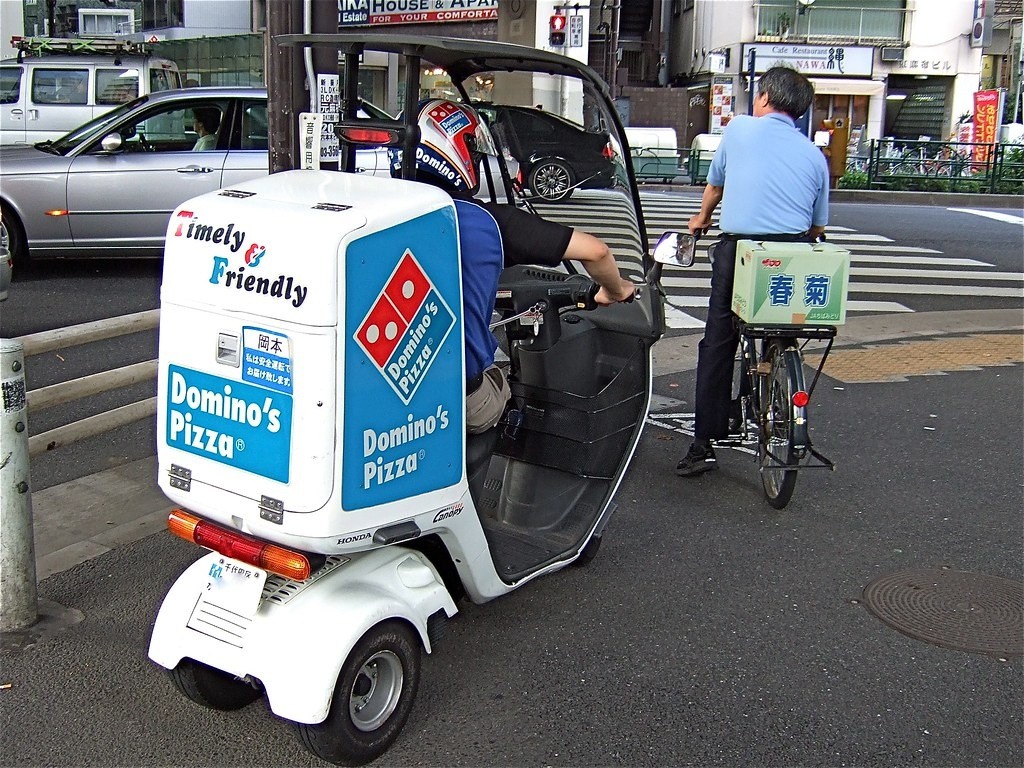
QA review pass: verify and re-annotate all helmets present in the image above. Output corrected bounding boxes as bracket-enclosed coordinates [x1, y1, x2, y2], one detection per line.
[387, 97, 499, 190]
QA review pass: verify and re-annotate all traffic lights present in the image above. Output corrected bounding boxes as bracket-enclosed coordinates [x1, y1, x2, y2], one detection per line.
[549, 15, 567, 48]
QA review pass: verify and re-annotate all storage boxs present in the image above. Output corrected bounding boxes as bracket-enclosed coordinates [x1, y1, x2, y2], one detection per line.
[731, 239, 851, 324]
[158, 167, 466, 554]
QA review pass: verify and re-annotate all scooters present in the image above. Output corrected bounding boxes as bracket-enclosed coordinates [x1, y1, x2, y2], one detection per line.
[145, 32, 696, 768]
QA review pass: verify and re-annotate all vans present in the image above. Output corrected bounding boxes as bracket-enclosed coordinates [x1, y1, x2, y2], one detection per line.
[0, 53, 184, 147]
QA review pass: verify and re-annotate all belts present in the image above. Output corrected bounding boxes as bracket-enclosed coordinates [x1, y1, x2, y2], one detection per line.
[719, 233, 804, 242]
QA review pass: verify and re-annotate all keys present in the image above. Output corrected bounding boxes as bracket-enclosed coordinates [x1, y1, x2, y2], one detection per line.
[528, 301, 548, 338]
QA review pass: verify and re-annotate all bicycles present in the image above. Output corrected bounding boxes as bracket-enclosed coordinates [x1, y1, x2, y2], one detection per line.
[692, 224, 837, 510]
[846, 137, 983, 178]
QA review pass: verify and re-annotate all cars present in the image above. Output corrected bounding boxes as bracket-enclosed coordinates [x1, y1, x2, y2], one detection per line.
[1, 86, 522, 285]
[466, 100, 619, 204]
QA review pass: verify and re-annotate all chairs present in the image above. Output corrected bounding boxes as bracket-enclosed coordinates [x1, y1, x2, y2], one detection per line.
[242, 111, 252, 149]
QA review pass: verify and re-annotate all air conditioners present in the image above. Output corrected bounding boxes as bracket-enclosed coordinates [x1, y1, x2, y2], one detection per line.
[882, 47, 905, 61]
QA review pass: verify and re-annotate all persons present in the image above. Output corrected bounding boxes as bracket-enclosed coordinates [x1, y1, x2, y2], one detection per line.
[676, 67, 830, 477]
[386, 98, 637, 433]
[189, 107, 220, 150]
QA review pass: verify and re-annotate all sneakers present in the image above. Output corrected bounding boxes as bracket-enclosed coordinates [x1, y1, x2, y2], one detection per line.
[676, 444, 717, 477]
